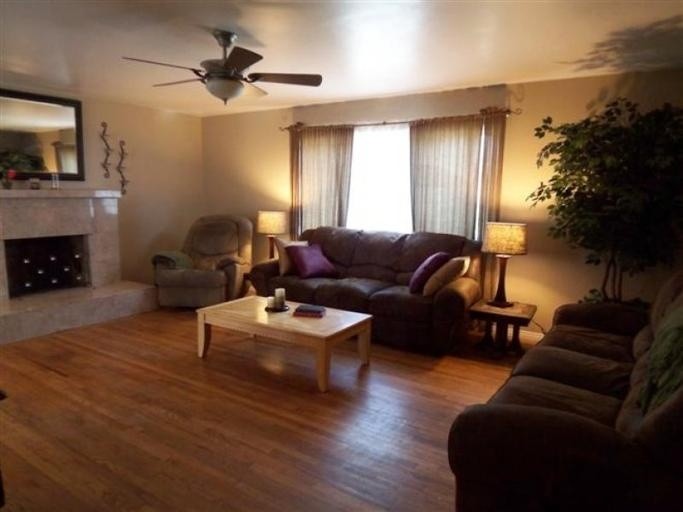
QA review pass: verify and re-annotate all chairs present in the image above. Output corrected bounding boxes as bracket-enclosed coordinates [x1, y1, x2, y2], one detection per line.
[153, 214, 253, 308]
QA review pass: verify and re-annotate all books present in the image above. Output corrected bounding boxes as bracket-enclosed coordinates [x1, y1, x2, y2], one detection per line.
[294, 304, 326, 318]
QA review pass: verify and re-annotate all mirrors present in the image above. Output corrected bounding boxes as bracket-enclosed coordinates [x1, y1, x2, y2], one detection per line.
[1, 88, 83, 181]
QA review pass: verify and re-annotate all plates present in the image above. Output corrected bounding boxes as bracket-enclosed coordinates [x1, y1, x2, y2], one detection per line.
[265, 306, 289, 313]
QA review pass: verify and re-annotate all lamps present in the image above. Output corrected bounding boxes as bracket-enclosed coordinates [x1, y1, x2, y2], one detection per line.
[256, 211, 287, 258]
[482, 222, 530, 305]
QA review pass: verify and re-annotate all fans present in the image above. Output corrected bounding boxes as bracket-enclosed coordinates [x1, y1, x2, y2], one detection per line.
[118, 31, 323, 106]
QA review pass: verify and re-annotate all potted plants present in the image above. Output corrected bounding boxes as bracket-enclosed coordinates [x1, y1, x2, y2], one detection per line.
[534, 97, 681, 307]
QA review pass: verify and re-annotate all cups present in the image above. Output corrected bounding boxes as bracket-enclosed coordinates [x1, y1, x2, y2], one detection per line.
[275, 288, 285, 309]
[268, 296, 274, 308]
[51, 173, 59, 189]
[30, 178, 40, 190]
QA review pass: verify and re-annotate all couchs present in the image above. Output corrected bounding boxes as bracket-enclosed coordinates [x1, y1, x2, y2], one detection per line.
[449, 270, 683, 512]
[248, 227, 482, 357]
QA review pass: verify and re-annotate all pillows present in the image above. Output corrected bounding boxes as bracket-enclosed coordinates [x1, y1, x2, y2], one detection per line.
[408, 252, 450, 294]
[274, 237, 307, 276]
[288, 244, 333, 278]
[424, 257, 470, 294]
